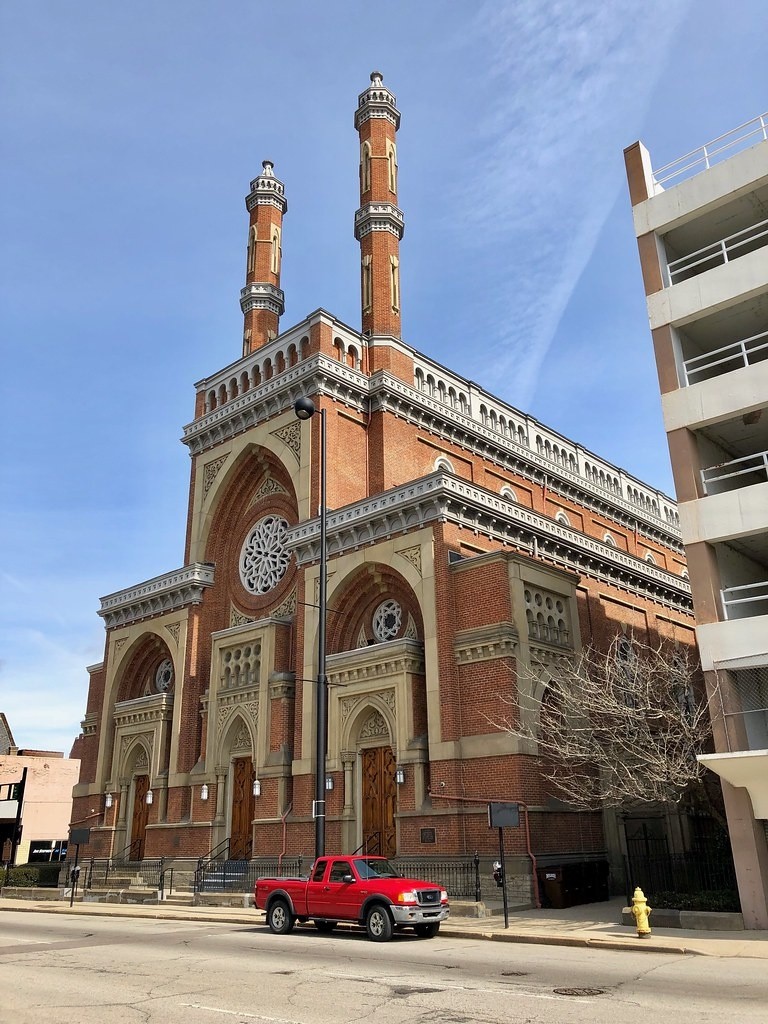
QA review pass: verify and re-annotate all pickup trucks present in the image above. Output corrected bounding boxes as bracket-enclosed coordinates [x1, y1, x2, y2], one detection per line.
[253, 854, 451, 940]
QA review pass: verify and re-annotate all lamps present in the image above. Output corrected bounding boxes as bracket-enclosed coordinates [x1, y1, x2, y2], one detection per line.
[396, 765, 404, 783]
[200, 784, 209, 800]
[105, 793, 113, 808]
[145, 789, 153, 805]
[253, 779, 261, 797]
[325, 776, 334, 790]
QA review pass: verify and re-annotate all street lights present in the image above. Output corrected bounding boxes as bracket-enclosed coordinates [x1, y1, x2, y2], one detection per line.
[292, 398, 330, 855]
